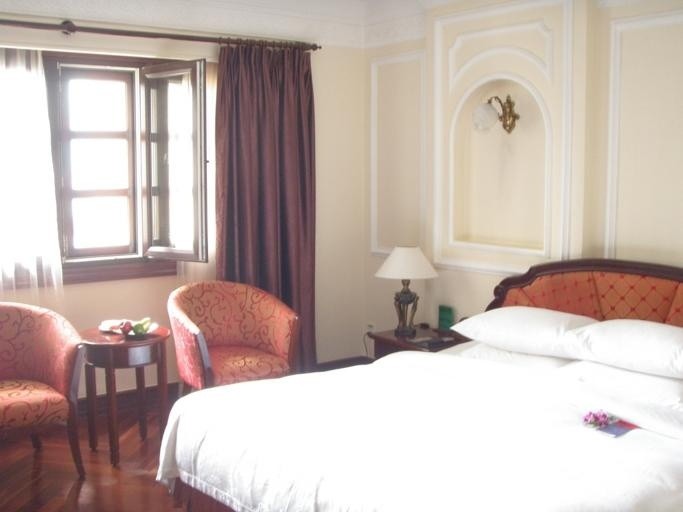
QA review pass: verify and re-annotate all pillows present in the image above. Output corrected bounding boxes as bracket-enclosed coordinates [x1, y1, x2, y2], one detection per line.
[565, 318, 683, 379]
[450, 305, 600, 359]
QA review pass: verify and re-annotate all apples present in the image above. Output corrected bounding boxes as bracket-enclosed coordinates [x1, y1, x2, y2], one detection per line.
[119, 321, 132, 334]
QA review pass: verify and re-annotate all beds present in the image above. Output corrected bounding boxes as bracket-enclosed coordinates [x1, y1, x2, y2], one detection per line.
[156, 258, 683, 512]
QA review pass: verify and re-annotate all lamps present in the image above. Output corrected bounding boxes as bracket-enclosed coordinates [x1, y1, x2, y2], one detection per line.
[374, 247, 440, 337]
[474, 94, 520, 134]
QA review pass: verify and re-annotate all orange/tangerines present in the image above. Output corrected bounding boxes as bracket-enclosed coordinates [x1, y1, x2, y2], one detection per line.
[132, 324, 145, 336]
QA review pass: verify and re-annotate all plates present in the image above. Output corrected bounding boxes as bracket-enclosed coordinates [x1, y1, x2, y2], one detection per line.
[114, 323, 158, 335]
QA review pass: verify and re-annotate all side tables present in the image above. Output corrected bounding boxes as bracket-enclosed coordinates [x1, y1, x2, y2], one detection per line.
[81, 326, 171, 466]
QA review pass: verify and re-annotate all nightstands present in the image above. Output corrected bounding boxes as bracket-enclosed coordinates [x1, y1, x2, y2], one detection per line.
[373, 324, 472, 360]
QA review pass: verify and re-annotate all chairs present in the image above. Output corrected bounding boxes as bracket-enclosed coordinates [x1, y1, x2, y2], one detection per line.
[0, 301, 86, 478]
[166, 280, 300, 397]
